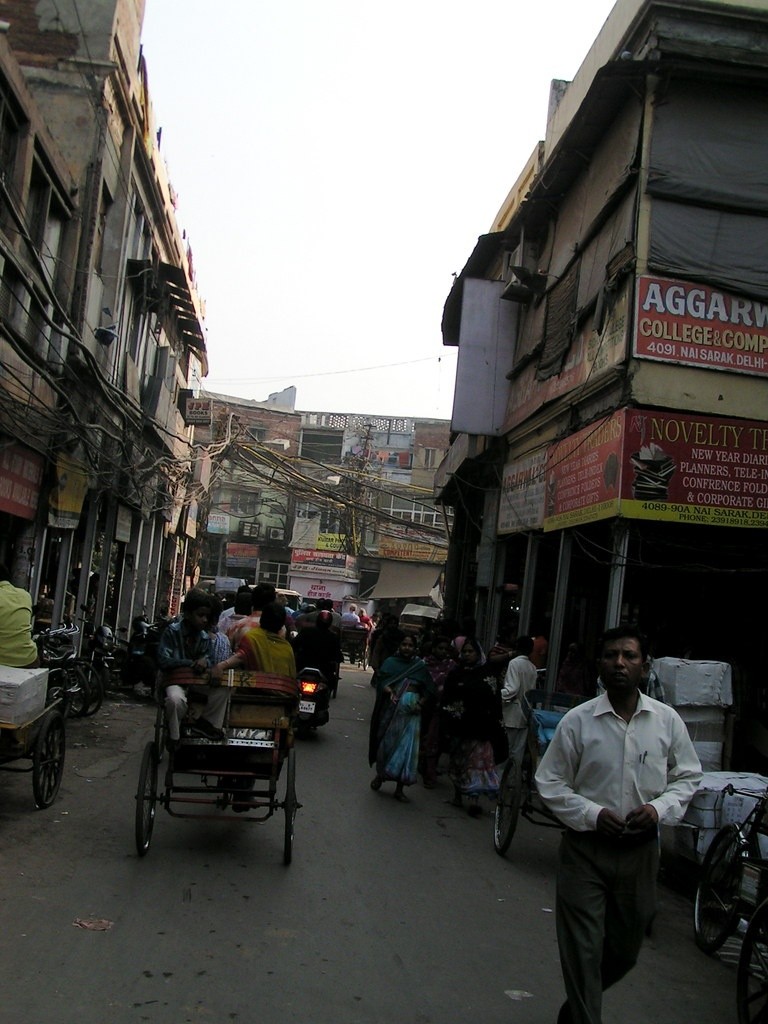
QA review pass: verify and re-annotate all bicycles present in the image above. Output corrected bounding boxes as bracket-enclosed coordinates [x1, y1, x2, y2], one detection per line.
[696, 782, 767, 1024]
[32, 616, 107, 720]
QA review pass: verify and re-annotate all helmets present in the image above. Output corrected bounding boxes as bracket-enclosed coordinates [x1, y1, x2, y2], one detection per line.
[316, 610, 333, 627]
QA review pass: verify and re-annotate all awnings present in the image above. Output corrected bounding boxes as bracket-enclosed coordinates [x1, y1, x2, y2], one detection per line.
[368, 557, 443, 599]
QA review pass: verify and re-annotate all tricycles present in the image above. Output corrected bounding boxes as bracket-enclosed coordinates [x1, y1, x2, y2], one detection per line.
[494, 689, 597, 858]
[339, 620, 371, 672]
[133, 662, 304, 866]
[0, 689, 80, 811]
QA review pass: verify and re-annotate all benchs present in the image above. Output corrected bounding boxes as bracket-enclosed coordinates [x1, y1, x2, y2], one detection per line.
[534, 708, 566, 751]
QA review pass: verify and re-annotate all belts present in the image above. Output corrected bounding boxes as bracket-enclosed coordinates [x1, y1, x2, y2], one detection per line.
[592, 827, 657, 850]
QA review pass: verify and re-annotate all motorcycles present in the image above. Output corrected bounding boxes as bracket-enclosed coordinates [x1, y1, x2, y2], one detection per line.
[288, 629, 342, 738]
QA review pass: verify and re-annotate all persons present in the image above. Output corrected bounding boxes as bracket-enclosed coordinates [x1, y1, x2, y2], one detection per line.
[341, 596, 537, 807]
[148, 581, 340, 796]
[534, 627, 704, 1024]
[0, 560, 44, 670]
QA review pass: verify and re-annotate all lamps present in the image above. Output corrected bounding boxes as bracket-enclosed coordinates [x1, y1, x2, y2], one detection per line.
[94, 323, 120, 347]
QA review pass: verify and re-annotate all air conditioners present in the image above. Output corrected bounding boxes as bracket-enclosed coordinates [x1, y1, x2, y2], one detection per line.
[504, 242, 540, 294]
[242, 523, 259, 538]
[268, 528, 285, 540]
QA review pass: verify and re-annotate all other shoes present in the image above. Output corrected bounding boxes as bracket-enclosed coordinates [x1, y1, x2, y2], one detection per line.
[165, 735, 182, 746]
[424, 783, 435, 788]
[468, 806, 482, 817]
[450, 799, 464, 807]
[371, 779, 381, 790]
[418, 767, 442, 776]
[394, 791, 410, 802]
[191, 716, 224, 740]
